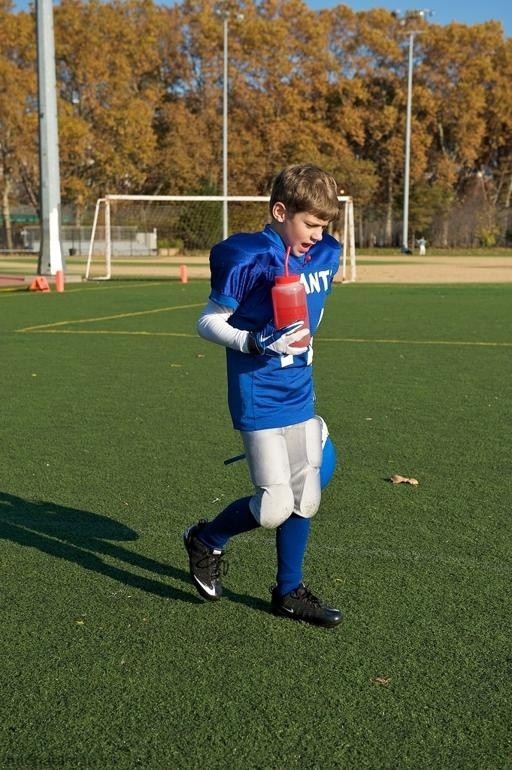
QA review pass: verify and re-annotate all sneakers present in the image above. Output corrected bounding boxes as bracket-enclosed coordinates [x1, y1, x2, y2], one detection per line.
[183, 519, 226, 602]
[269, 582, 344, 629]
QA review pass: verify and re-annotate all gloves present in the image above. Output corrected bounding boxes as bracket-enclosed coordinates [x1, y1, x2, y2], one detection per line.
[246, 318, 310, 357]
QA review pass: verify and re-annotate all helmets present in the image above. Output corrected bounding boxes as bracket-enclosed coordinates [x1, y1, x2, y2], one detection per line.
[315, 414, 337, 493]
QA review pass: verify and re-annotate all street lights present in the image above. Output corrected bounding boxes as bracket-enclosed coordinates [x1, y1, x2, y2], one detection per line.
[391, 9, 435, 249]
[208, 8, 245, 243]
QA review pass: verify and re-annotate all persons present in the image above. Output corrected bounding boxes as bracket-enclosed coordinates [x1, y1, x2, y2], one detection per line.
[183, 162, 346, 630]
[416, 236, 429, 256]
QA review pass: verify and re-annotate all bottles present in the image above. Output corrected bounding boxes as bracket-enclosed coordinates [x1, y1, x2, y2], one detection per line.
[270, 273, 312, 349]
